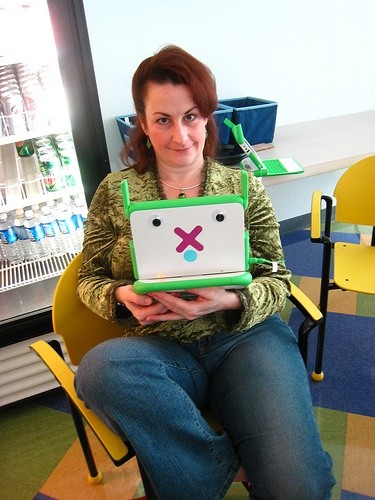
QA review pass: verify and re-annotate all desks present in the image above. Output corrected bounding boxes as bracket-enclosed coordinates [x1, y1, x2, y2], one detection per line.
[257, 110, 375, 187]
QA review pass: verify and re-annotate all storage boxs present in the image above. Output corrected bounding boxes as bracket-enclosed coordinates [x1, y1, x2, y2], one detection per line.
[115, 97, 278, 160]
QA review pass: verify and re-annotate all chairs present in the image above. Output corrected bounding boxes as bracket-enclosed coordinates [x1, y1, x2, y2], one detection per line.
[29, 251, 323, 485]
[310, 155, 375, 381]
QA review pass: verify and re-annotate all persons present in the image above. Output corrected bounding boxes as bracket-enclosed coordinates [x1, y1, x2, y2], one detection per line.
[73, 45, 336, 500]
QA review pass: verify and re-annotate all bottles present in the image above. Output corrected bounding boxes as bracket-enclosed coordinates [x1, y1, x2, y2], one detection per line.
[0, 195, 88, 265]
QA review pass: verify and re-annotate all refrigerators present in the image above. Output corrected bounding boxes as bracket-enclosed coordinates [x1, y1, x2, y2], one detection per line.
[0, 0, 113, 408]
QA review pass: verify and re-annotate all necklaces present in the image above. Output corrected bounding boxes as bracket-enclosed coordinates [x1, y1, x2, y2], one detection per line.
[159, 178, 206, 199]
